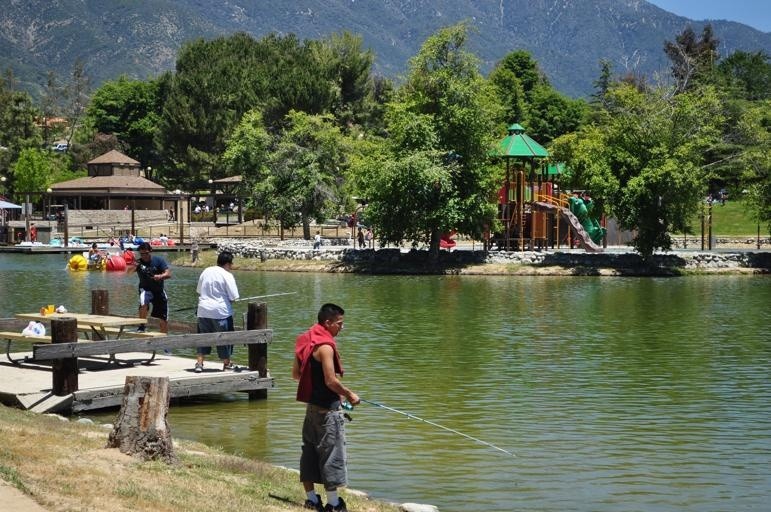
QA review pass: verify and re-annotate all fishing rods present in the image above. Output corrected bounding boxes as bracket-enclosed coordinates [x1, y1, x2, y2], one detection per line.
[63, 197, 154, 278]
[168, 290, 299, 312]
[359, 397, 532, 466]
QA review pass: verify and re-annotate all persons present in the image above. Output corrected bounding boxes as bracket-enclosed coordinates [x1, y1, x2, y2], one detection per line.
[292, 302, 361, 512]
[193, 248, 243, 373]
[125, 242, 173, 354]
[195, 204, 202, 216]
[357, 227, 367, 249]
[168, 206, 175, 222]
[202, 202, 210, 214]
[30, 222, 37, 244]
[230, 201, 236, 211]
[90, 243, 103, 258]
[109, 226, 170, 252]
[313, 230, 322, 249]
[365, 228, 374, 248]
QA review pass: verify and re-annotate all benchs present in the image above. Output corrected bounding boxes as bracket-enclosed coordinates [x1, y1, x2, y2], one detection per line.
[76, 324, 168, 366]
[0, 327, 100, 373]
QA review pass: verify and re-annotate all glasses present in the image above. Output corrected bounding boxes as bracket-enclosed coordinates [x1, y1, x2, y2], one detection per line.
[138, 251, 150, 257]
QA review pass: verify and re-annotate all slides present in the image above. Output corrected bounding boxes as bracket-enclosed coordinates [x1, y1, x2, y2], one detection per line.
[531, 197, 607, 253]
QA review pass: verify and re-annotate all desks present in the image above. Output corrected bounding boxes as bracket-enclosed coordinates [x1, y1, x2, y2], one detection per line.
[13, 307, 148, 372]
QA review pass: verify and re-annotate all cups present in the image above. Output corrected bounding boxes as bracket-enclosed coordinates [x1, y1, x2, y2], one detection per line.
[48, 305, 55, 312]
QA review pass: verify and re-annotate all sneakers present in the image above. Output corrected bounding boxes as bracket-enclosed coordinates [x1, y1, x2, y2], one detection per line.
[137, 324, 149, 333]
[325, 497, 348, 512]
[304, 494, 324, 511]
[164, 348, 172, 356]
[194, 361, 203, 372]
[223, 362, 239, 372]
